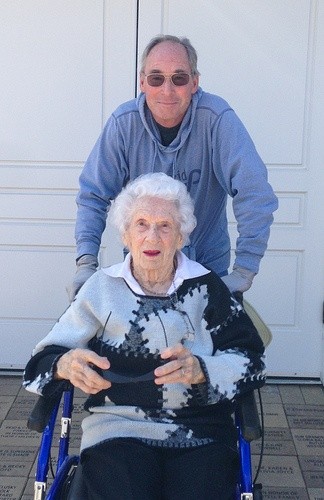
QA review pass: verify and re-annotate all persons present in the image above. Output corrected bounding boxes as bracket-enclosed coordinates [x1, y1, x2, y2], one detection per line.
[22, 171, 268, 500]
[73, 34, 279, 308]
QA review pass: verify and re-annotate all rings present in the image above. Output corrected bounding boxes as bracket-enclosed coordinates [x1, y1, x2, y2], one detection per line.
[181, 366, 186, 376]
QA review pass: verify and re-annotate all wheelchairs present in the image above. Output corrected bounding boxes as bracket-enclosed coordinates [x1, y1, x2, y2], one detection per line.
[26, 374, 266, 500]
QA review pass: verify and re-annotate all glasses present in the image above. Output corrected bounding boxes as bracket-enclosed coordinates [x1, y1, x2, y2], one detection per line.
[143, 70, 193, 88]
